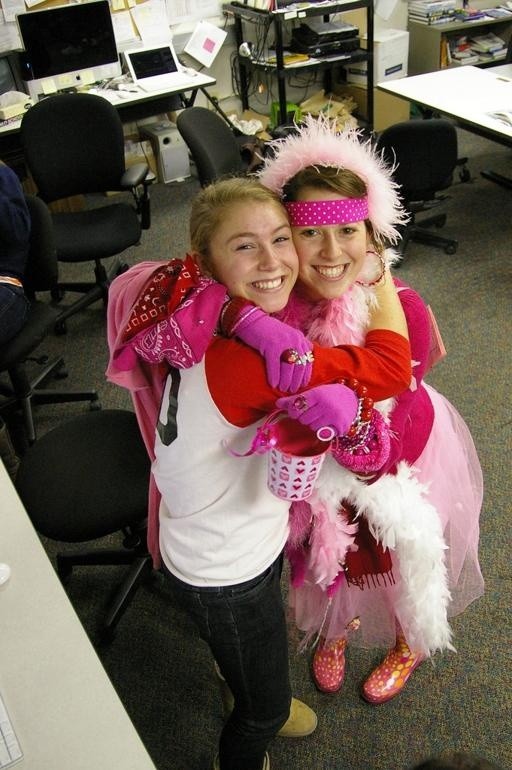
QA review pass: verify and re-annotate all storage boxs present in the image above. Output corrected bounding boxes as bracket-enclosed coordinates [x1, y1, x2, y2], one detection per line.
[333, 28, 410, 136]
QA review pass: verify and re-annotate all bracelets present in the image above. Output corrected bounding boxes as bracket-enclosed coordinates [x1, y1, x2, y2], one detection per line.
[228, 308, 264, 335]
[322, 376, 375, 451]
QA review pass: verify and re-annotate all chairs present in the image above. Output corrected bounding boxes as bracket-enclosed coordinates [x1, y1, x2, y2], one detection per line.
[2, 409, 169, 645]
[177, 107, 249, 189]
[20, 93, 156, 335]
[0, 196, 102, 448]
[377, 119, 457, 269]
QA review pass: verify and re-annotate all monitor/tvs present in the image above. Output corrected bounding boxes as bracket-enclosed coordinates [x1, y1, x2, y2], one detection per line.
[0, 51, 27, 97]
[14, 0, 123, 99]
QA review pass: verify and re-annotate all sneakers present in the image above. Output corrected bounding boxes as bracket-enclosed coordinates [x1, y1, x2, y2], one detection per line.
[218, 680, 320, 740]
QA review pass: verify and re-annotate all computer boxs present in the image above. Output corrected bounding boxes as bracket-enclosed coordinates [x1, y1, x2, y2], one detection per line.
[138, 119, 192, 185]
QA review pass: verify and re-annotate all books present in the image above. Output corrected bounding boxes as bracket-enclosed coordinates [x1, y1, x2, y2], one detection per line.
[408, 1, 511, 64]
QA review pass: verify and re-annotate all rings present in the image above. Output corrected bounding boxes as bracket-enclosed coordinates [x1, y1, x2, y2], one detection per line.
[292, 394, 306, 411]
[280, 348, 299, 364]
[317, 426, 335, 442]
[297, 350, 314, 366]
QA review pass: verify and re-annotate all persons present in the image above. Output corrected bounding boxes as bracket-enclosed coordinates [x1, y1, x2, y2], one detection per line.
[107, 179, 412, 769]
[108, 168, 451, 702]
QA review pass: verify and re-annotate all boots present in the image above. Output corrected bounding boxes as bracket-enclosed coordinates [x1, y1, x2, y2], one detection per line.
[311, 622, 352, 696]
[361, 607, 430, 707]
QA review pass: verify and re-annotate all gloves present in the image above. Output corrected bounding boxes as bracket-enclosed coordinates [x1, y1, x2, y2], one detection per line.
[231, 305, 319, 394]
[275, 382, 359, 443]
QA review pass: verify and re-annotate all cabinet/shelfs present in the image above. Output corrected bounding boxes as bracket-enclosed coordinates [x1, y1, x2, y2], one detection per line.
[223, 0, 374, 144]
[407, 14, 512, 118]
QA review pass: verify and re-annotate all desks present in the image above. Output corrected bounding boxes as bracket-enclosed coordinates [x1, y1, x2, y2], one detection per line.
[0, 461, 160, 770]
[0, 65, 240, 191]
[374, 66, 512, 192]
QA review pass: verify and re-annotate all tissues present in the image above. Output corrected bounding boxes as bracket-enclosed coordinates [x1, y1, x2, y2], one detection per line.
[0, 90, 35, 121]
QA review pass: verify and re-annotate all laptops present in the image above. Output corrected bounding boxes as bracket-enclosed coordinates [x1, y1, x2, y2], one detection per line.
[124, 42, 193, 93]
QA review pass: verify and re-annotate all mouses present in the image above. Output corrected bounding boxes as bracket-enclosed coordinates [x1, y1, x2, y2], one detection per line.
[185, 67, 198, 77]
[117, 91, 129, 99]
